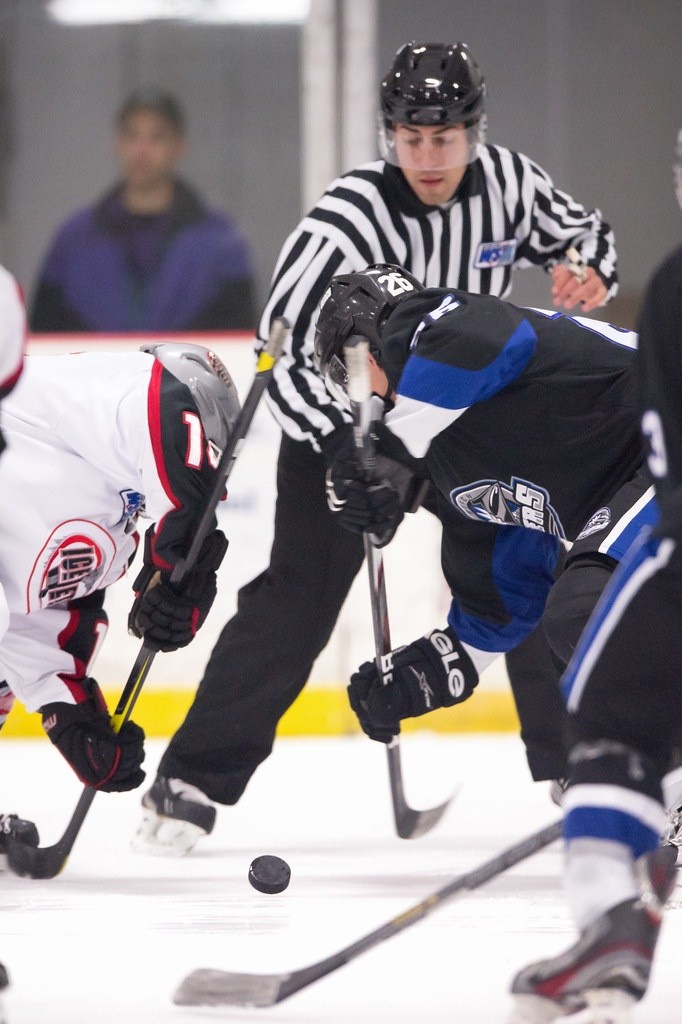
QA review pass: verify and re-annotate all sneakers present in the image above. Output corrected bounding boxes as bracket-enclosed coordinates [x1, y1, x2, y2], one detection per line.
[507, 895, 660, 1024]
[132, 777, 218, 859]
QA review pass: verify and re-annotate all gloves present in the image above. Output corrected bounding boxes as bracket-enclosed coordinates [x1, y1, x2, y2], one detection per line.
[39, 703, 146, 794]
[346, 628, 479, 743]
[321, 443, 403, 545]
[126, 525, 229, 653]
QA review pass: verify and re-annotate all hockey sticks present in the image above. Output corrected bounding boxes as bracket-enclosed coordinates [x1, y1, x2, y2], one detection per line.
[340, 331, 464, 841]
[0, 311, 295, 883]
[171, 813, 568, 1010]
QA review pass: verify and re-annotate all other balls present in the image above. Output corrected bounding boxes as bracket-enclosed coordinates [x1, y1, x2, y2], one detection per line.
[248, 854, 292, 894]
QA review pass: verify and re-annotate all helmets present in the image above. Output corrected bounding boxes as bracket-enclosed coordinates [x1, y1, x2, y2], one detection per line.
[313, 264, 422, 404]
[148, 339, 243, 478]
[381, 41, 486, 131]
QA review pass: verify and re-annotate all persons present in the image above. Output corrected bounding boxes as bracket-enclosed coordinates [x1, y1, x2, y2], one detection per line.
[30, 87, 251, 337]
[1, 142, 682, 1023]
[131, 36, 620, 859]
